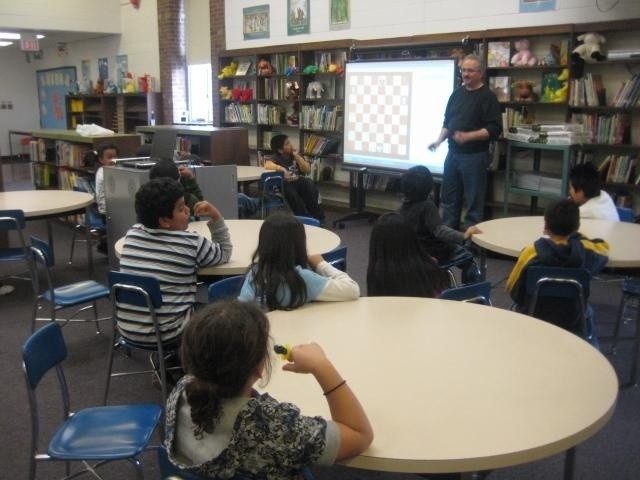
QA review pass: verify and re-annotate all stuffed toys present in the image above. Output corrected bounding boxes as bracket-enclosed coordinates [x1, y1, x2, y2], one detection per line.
[537, 44, 561, 65]
[63, 72, 155, 95]
[509, 37, 537, 66]
[217, 54, 345, 101]
[570, 31, 608, 62]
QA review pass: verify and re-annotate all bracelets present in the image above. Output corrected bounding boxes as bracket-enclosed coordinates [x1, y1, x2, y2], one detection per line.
[323, 379, 348, 398]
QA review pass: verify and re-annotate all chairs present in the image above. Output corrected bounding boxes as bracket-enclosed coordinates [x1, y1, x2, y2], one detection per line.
[524, 263, 600, 351]
[185, 166, 239, 221]
[208, 274, 245, 306]
[68, 185, 108, 267]
[101, 166, 150, 255]
[15, 322, 163, 480]
[1, 209, 52, 300]
[318, 246, 348, 274]
[259, 171, 286, 220]
[100, 268, 178, 406]
[440, 281, 491, 306]
[611, 277, 639, 352]
[292, 215, 322, 228]
[26, 235, 111, 343]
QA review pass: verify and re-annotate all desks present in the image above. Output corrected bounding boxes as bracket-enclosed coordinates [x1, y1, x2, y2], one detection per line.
[184, 218, 342, 276]
[2, 190, 96, 220]
[8, 129, 141, 190]
[251, 293, 620, 474]
[470, 215, 640, 269]
[237, 165, 276, 182]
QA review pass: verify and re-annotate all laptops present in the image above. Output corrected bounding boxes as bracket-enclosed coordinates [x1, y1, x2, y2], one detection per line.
[122, 126, 178, 169]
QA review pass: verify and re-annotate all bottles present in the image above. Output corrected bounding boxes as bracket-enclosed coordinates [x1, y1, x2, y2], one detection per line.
[180, 112, 186, 124]
[150, 110, 155, 126]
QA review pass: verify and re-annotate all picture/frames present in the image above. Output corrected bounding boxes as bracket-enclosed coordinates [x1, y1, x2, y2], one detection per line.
[329, 2, 352, 29]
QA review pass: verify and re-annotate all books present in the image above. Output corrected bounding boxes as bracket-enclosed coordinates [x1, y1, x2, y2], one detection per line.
[607, 49, 640, 61]
[484, 67, 640, 193]
[26, 137, 91, 232]
[223, 102, 344, 183]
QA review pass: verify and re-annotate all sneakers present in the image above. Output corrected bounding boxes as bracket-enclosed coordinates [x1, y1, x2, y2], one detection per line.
[153, 370, 176, 394]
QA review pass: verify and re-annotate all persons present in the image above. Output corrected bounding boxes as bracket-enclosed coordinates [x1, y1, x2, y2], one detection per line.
[82, 142, 122, 256]
[506, 196, 613, 351]
[567, 160, 621, 222]
[263, 134, 325, 218]
[163, 297, 375, 480]
[149, 157, 204, 223]
[237, 211, 362, 314]
[427, 53, 504, 233]
[291, 5, 305, 25]
[398, 164, 480, 285]
[365, 210, 446, 300]
[248, 13, 268, 32]
[113, 176, 233, 393]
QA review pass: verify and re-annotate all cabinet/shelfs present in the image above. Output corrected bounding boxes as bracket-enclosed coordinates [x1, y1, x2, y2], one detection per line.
[219, 41, 348, 191]
[480, 19, 640, 224]
[65, 92, 164, 135]
[133, 123, 249, 165]
